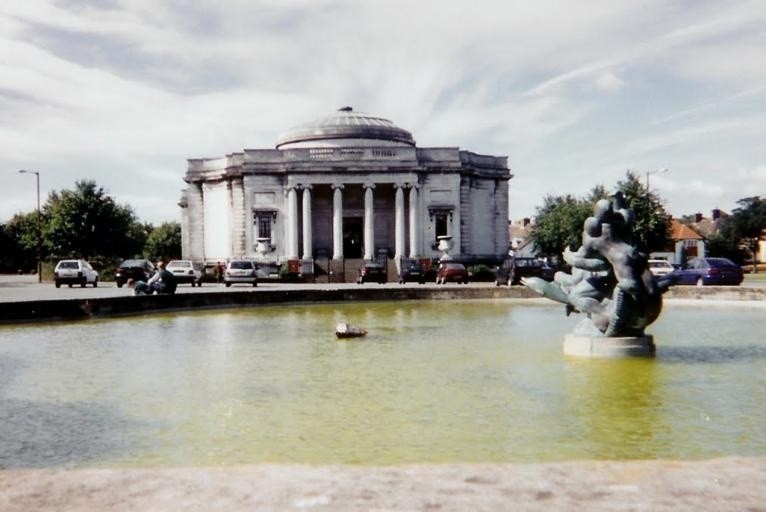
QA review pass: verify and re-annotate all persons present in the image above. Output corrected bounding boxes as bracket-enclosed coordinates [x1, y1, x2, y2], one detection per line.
[147, 261, 178, 295]
[214, 260, 223, 283]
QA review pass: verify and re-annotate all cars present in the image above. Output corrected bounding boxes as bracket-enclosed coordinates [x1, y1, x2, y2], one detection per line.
[538, 258, 556, 282]
[434, 262, 471, 285]
[114, 257, 156, 289]
[397, 263, 426, 285]
[165, 258, 204, 288]
[648, 259, 675, 278]
[358, 262, 384, 284]
[223, 258, 261, 287]
[665, 257, 746, 285]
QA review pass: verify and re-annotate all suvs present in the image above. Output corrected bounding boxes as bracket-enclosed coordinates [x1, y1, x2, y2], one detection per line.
[51, 258, 100, 288]
[494, 256, 542, 288]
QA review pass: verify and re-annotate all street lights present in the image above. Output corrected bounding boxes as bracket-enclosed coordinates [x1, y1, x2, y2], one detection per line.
[753, 237, 759, 273]
[17, 168, 43, 283]
[646, 167, 670, 262]
